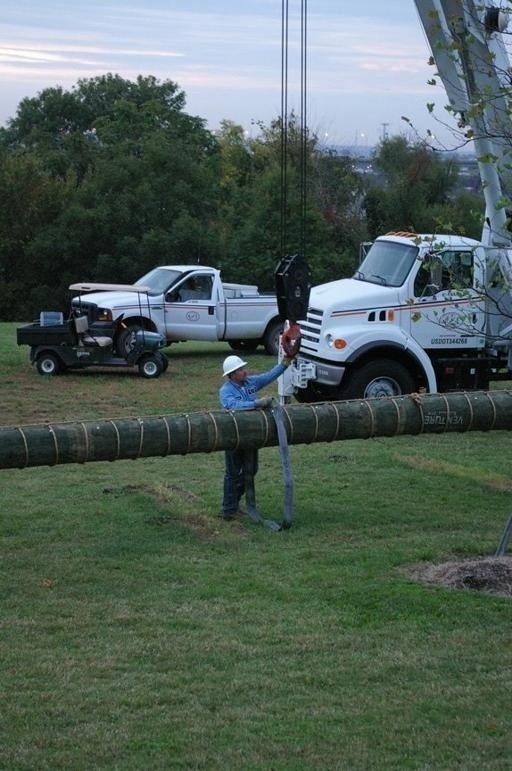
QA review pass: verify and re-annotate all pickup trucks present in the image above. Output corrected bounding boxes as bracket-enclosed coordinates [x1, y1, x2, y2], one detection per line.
[69, 263, 289, 357]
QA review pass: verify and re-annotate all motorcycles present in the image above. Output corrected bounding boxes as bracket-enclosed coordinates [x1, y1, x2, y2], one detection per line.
[16, 281, 168, 379]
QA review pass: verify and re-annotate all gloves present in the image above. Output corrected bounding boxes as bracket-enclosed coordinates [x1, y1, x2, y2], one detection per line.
[282, 356, 291, 366]
[253, 397, 278, 408]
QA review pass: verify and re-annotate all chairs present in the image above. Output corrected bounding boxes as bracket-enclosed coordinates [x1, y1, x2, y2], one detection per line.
[74, 315, 114, 347]
[177, 289, 209, 303]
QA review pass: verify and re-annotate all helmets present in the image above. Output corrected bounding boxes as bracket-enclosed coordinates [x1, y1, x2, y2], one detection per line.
[222, 355, 248, 377]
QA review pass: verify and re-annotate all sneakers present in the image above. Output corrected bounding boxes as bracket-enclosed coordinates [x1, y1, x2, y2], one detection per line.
[222, 508, 250, 521]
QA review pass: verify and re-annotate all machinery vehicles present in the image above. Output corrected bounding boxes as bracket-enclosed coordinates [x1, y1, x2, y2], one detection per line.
[274, 0, 512, 403]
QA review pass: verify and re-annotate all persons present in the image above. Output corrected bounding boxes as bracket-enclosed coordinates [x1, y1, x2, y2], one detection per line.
[217, 352, 295, 523]
